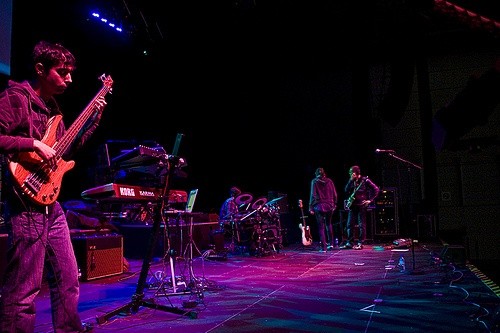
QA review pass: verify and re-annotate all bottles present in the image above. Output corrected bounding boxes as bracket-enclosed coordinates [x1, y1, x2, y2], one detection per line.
[399, 256, 405, 272]
[195, 279, 203, 301]
[334, 236, 338, 250]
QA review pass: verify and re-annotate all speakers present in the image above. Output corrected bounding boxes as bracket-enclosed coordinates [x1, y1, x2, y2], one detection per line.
[334, 210, 374, 243]
[71, 235, 124, 280]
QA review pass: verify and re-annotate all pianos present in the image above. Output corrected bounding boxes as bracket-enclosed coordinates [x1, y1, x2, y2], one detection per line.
[80, 145, 199, 330]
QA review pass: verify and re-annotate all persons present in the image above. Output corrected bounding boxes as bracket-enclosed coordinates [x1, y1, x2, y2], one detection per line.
[0, 41, 107, 333]
[345, 166, 379, 250]
[309, 168, 338, 252]
[220, 187, 241, 219]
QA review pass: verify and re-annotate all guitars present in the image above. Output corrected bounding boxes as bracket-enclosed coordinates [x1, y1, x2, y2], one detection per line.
[4, 72, 114, 210]
[297, 199, 313, 246]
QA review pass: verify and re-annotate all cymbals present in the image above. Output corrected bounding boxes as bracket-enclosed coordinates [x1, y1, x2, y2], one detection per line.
[234, 193, 254, 206]
[252, 197, 269, 209]
[266, 193, 286, 205]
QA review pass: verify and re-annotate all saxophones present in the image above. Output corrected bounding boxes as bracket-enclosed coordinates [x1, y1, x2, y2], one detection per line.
[344, 176, 368, 210]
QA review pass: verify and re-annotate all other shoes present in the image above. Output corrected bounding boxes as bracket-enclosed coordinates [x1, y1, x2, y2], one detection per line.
[319, 249, 327, 253]
[352, 243, 362, 250]
[327, 245, 334, 250]
[339, 243, 352, 248]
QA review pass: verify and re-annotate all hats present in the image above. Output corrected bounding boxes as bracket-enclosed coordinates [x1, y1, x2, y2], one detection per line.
[230, 187, 241, 197]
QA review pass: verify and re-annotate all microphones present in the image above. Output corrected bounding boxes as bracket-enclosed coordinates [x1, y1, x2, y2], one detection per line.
[91, 252, 96, 268]
[376, 149, 394, 153]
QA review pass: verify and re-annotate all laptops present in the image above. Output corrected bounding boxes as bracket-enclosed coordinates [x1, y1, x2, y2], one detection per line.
[164, 189, 198, 213]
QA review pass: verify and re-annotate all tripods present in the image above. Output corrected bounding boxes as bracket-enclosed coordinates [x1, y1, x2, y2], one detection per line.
[295, 244, 318, 251]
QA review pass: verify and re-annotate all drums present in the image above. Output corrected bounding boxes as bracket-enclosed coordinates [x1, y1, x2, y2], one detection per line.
[240, 216, 282, 252]
